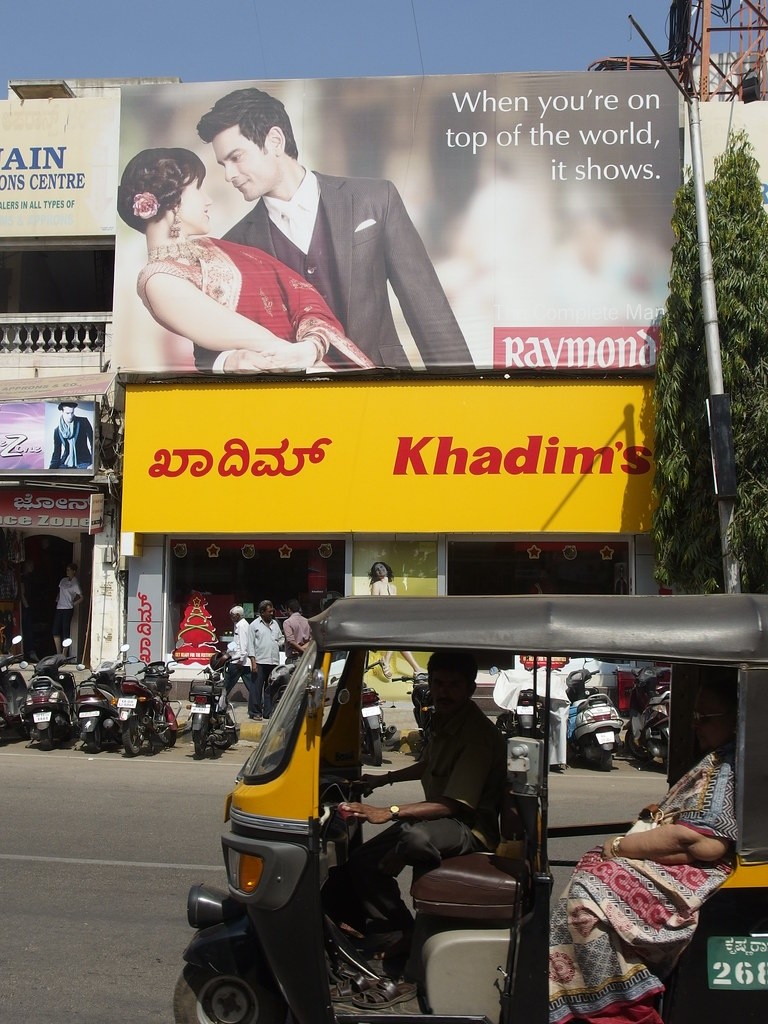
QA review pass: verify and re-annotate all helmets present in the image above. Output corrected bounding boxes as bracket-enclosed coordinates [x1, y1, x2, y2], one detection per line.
[210, 652, 232, 673]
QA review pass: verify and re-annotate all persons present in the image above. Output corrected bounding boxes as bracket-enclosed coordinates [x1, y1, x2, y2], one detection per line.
[48, 402, 94, 470]
[367, 562, 427, 680]
[330, 651, 507, 1010]
[117, 89, 481, 374]
[21, 559, 40, 662]
[51, 563, 85, 657]
[548, 679, 740, 1024]
[222, 600, 312, 721]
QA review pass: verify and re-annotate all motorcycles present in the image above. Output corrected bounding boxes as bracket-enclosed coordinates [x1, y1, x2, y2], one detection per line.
[621, 665, 673, 768]
[186, 657, 242, 760]
[262, 651, 303, 719]
[174, 590, 768, 1024]
[488, 665, 545, 773]
[553, 658, 627, 771]
[330, 661, 397, 766]
[391, 672, 436, 752]
[0, 634, 181, 757]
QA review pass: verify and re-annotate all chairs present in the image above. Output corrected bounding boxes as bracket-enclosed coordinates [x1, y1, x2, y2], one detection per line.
[412, 786, 541, 914]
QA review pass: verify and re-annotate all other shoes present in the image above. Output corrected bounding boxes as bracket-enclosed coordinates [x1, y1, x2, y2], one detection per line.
[250, 715, 262, 720]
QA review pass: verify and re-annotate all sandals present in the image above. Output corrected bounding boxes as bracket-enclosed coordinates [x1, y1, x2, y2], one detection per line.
[414, 671, 429, 678]
[379, 657, 392, 679]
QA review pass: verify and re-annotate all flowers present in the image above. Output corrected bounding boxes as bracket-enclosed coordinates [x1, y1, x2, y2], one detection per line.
[132, 191, 159, 219]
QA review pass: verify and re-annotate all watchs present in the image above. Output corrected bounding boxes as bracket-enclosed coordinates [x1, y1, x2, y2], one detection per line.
[389, 805, 399, 821]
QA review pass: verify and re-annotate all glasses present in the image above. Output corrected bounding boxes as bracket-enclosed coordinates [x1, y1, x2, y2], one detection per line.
[693, 709, 726, 723]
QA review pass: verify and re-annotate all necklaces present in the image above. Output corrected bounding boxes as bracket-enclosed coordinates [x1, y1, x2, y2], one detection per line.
[146, 241, 215, 262]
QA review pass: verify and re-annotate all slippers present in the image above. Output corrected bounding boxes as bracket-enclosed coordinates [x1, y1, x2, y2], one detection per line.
[352, 978, 417, 1008]
[330, 973, 381, 1001]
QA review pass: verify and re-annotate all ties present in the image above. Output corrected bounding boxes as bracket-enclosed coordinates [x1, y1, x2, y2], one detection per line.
[281, 202, 311, 256]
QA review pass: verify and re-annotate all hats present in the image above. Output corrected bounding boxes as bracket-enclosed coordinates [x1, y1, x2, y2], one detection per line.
[58, 401, 79, 410]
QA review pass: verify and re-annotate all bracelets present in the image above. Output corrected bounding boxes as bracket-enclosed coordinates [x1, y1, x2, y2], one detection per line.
[388, 770, 394, 785]
[612, 837, 624, 857]
[300, 337, 324, 363]
